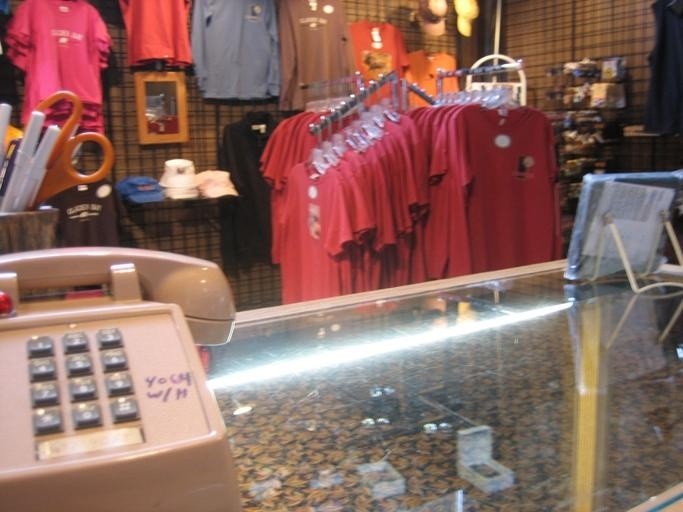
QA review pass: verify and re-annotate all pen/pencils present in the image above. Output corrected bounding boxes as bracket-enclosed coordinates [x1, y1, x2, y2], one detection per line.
[0, 103, 83, 212]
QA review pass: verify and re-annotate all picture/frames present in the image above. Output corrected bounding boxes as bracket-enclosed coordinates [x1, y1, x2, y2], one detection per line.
[132, 71, 189, 146]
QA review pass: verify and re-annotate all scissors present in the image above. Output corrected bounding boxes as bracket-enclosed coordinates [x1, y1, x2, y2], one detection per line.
[24, 90, 115, 212]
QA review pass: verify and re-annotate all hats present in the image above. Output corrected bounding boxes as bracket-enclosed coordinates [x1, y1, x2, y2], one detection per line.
[116, 158, 240, 204]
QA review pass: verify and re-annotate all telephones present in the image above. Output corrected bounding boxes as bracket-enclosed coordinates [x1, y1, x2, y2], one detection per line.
[0, 246, 242, 512]
